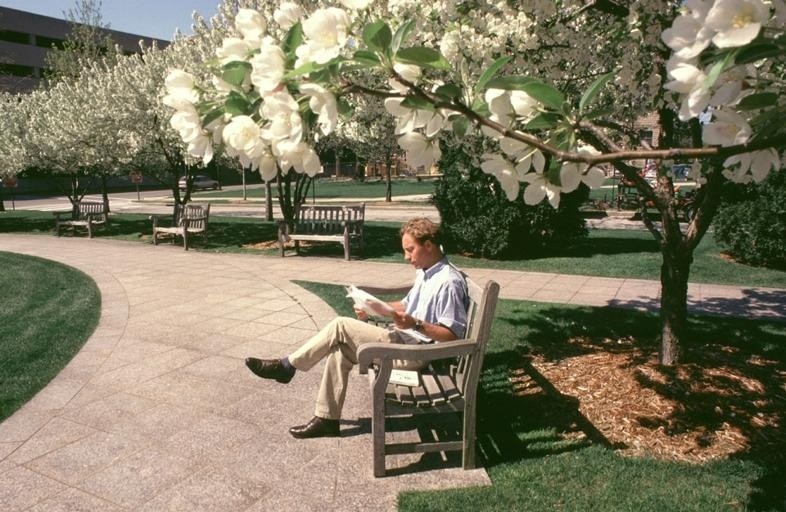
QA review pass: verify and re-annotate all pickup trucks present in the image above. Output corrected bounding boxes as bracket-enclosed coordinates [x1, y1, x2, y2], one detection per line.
[615, 163, 702, 223]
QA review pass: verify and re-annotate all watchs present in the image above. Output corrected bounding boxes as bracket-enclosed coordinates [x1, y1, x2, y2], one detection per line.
[413, 319, 424, 331]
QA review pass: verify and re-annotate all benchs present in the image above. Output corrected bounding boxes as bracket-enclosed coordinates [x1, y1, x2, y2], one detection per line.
[52, 200, 110, 239]
[150, 202, 213, 252]
[356, 244, 499, 479]
[275, 201, 367, 260]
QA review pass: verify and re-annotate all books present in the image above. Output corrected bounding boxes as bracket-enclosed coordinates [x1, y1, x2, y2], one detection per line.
[344, 282, 395, 321]
[387, 366, 420, 387]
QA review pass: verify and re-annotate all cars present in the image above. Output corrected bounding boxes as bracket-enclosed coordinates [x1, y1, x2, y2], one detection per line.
[178, 173, 219, 193]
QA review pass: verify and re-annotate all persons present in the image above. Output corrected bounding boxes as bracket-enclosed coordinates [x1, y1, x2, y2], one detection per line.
[245, 215, 470, 439]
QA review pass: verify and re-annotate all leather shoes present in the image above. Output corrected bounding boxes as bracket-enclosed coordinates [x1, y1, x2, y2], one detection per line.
[289, 417, 340, 438]
[246, 358, 294, 384]
